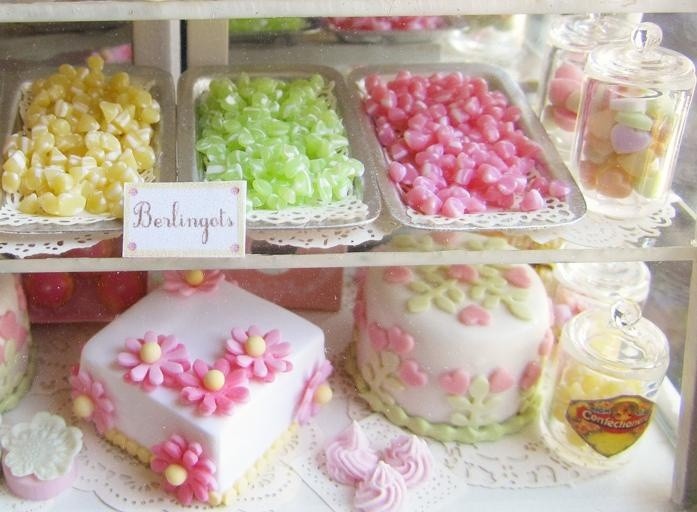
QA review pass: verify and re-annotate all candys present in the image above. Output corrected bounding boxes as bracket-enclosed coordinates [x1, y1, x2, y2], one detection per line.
[551, 266, 648, 448]
[546, 41, 676, 199]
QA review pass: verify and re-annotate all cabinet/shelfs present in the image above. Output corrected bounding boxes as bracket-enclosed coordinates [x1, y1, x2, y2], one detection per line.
[0, 0, 697, 512]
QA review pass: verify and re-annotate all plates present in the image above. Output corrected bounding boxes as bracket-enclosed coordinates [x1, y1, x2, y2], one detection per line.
[176, 62, 379, 233]
[322, 16, 470, 43]
[345, 59, 589, 235]
[228, 18, 324, 48]
[0, 60, 179, 239]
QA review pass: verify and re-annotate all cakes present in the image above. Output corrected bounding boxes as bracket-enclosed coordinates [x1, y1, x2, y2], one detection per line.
[67, 270, 332, 507]
[343, 230, 556, 444]
[0, 256, 37, 406]
[327, 419, 431, 512]
[0, 412, 83, 502]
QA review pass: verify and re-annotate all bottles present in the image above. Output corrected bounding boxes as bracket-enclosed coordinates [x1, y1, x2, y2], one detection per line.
[573, 20, 696, 219]
[536, 293, 673, 471]
[552, 241, 652, 313]
[535, 15, 640, 155]
